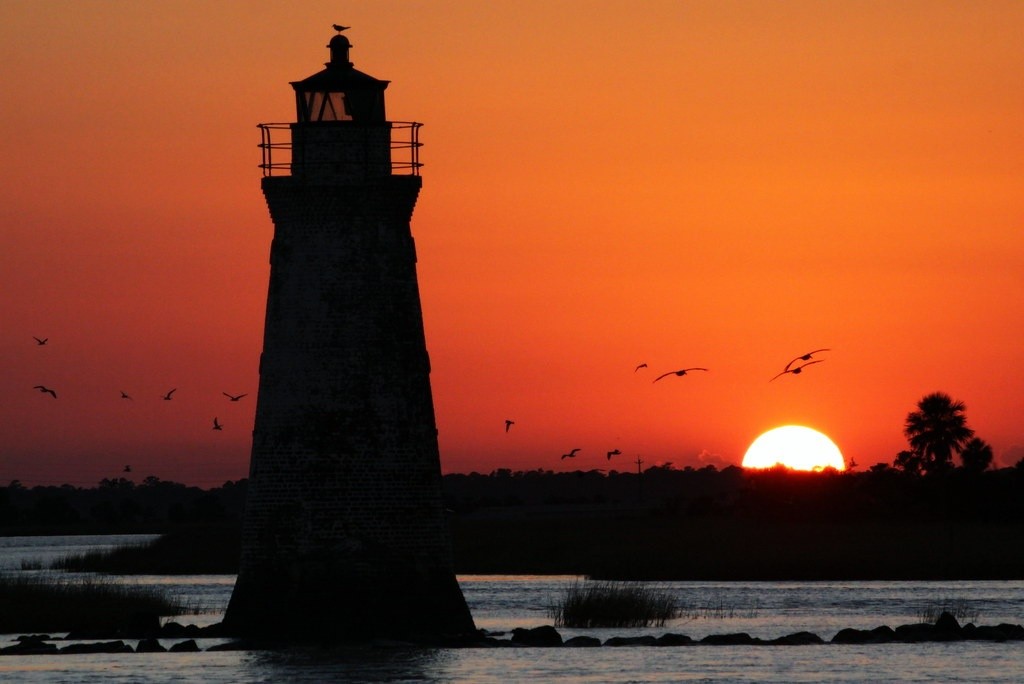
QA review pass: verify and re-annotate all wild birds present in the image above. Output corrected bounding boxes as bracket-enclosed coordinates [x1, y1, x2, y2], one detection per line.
[607, 449, 621, 460]
[160, 389, 176, 400]
[120, 390, 132, 400]
[34, 385, 57, 399]
[32, 337, 49, 345]
[635, 363, 707, 383]
[123, 464, 132, 474]
[212, 417, 223, 431]
[223, 392, 248, 401]
[770, 349, 830, 381]
[505, 420, 514, 432]
[561, 449, 581, 459]
